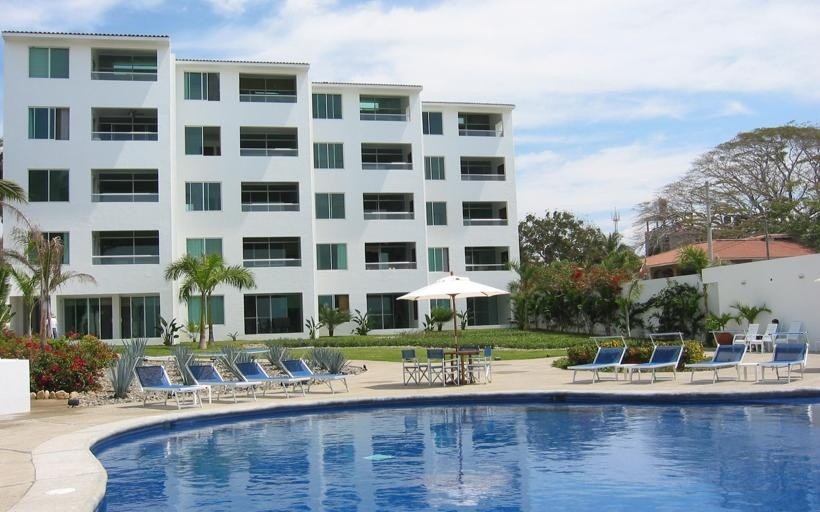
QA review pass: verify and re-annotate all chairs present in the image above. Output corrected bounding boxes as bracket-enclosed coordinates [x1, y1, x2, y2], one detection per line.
[566, 322, 809, 384]
[402, 345, 492, 387]
[133, 358, 349, 410]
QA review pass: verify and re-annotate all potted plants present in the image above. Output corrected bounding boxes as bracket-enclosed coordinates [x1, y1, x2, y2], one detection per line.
[729, 301, 772, 351]
[704, 310, 742, 345]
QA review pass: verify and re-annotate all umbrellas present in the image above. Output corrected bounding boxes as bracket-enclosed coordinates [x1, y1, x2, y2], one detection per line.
[395, 270, 511, 364]
